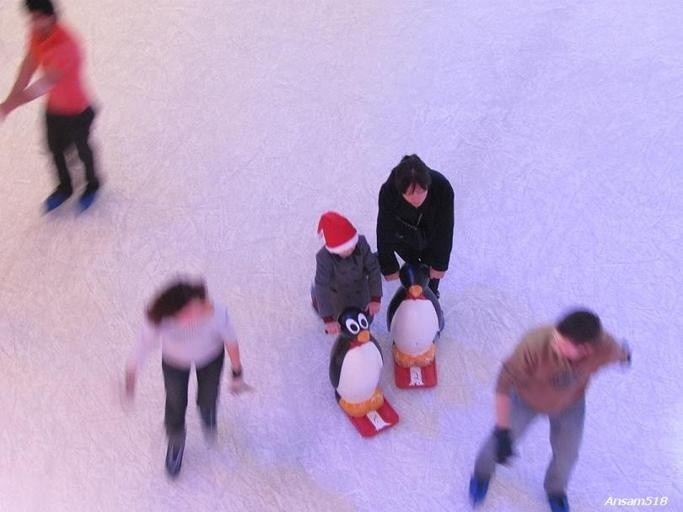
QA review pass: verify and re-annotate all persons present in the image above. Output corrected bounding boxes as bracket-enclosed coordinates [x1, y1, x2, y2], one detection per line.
[330, 306, 400, 436]
[0, 1, 100, 219]
[118, 272, 256, 479]
[308, 210, 382, 332]
[465, 307, 633, 512]
[375, 151, 454, 302]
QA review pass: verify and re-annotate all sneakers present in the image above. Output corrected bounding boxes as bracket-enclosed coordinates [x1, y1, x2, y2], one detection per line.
[470, 473, 489, 501]
[166, 429, 186, 477]
[203, 412, 216, 438]
[548, 492, 569, 512]
[47, 179, 99, 212]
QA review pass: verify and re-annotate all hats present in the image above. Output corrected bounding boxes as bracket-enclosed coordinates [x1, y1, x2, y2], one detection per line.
[318, 212, 359, 255]
